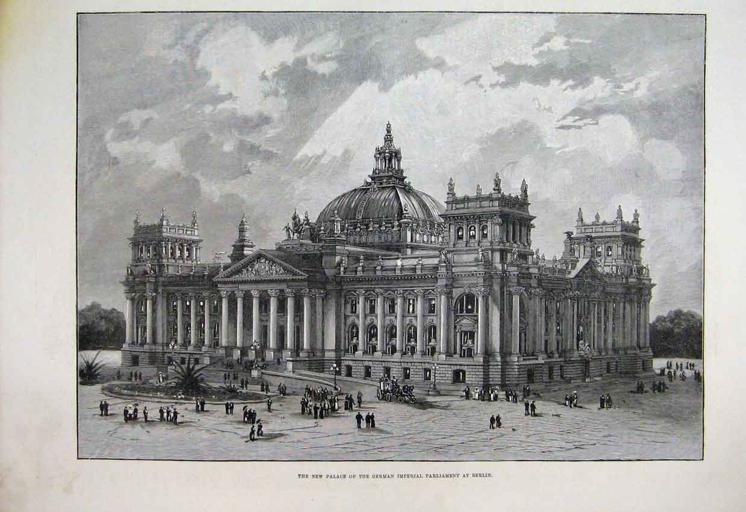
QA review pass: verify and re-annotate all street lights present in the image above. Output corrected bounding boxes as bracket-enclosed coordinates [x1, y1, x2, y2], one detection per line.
[329, 363, 339, 391]
[431, 363, 439, 389]
[249, 340, 260, 369]
